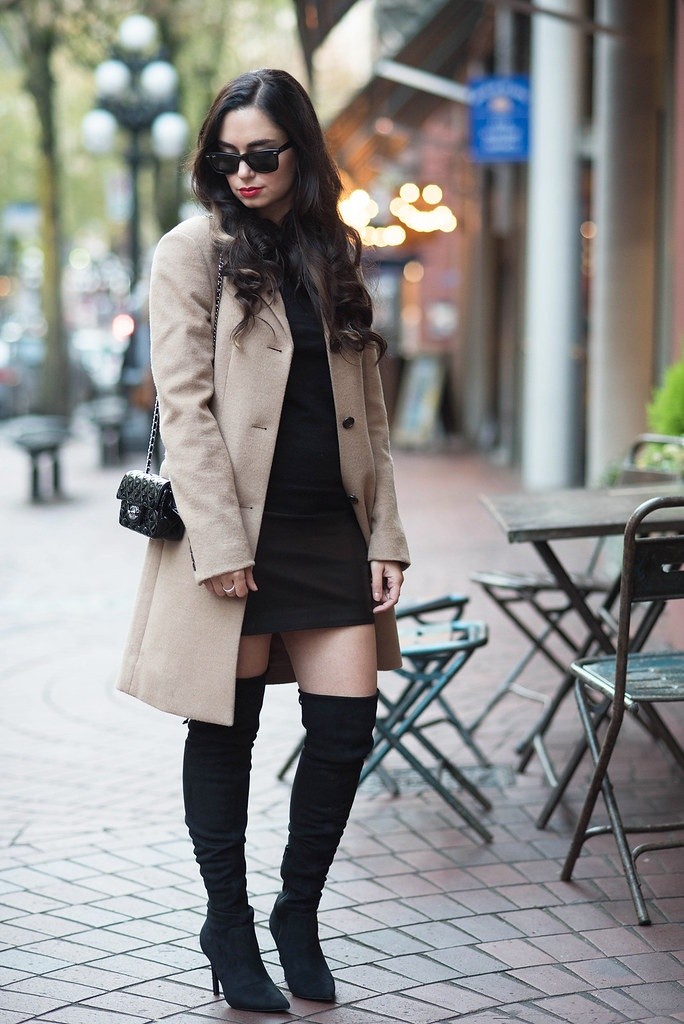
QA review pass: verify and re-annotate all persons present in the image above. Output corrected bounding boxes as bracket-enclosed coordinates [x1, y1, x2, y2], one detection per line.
[115, 68, 412, 1013]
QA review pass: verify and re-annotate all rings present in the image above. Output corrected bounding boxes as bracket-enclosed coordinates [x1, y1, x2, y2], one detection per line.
[222, 586, 235, 594]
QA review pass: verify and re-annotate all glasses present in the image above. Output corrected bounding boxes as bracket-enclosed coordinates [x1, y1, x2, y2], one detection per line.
[202, 136, 292, 173]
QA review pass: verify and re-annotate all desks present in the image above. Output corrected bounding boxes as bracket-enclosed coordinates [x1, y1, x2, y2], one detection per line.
[480, 482, 684, 831]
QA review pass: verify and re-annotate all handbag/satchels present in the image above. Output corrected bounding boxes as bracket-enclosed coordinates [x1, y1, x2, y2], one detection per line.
[116, 470, 184, 542]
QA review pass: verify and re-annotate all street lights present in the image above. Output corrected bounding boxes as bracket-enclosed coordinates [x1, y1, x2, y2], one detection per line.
[81, 14, 189, 398]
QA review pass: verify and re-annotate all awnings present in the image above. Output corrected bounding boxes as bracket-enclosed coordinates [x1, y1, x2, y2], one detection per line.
[311, 0, 496, 188]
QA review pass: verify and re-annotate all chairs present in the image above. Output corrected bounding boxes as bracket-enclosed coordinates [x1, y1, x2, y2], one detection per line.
[278, 441, 684, 926]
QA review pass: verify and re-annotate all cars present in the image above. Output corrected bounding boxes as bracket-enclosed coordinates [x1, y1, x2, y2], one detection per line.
[0, 325, 99, 422]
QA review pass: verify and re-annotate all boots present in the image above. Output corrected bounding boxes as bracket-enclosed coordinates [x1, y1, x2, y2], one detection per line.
[184, 671, 291, 1011]
[268, 684, 380, 999]
